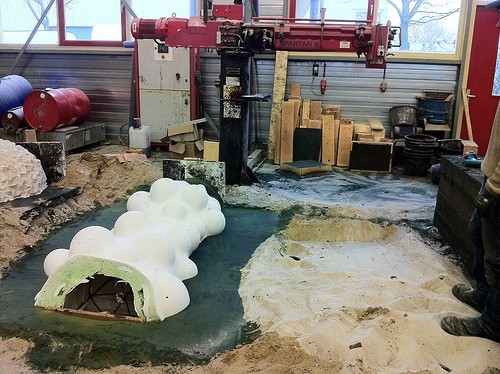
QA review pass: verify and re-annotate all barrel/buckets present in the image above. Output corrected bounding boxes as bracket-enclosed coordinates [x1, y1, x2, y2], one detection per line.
[404, 134, 463, 185]
[23, 88, 91, 132]
[0, 74, 33, 132]
[1, 106, 24, 132]
[129, 126, 151, 148]
[389, 96, 453, 126]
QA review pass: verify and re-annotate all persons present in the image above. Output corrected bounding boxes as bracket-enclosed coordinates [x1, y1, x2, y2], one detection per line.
[441, 99, 500, 343]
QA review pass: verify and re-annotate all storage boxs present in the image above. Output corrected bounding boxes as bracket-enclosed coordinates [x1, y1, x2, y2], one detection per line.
[38, 122, 105, 151]
[160, 124, 205, 159]
[433, 155, 485, 251]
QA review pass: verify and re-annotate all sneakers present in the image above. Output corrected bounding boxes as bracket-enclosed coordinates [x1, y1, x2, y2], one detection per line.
[441, 283, 496, 341]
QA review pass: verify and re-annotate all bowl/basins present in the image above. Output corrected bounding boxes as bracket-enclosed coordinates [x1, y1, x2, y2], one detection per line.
[424, 91, 452, 100]
